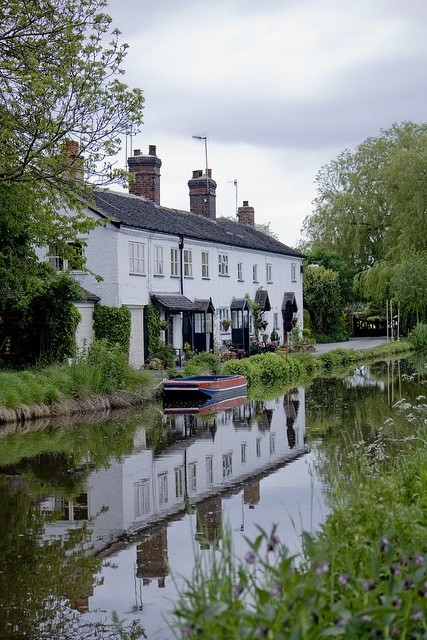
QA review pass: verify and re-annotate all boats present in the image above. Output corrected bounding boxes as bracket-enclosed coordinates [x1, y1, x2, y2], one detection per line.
[164, 375, 246, 398]
[162, 392, 247, 415]
